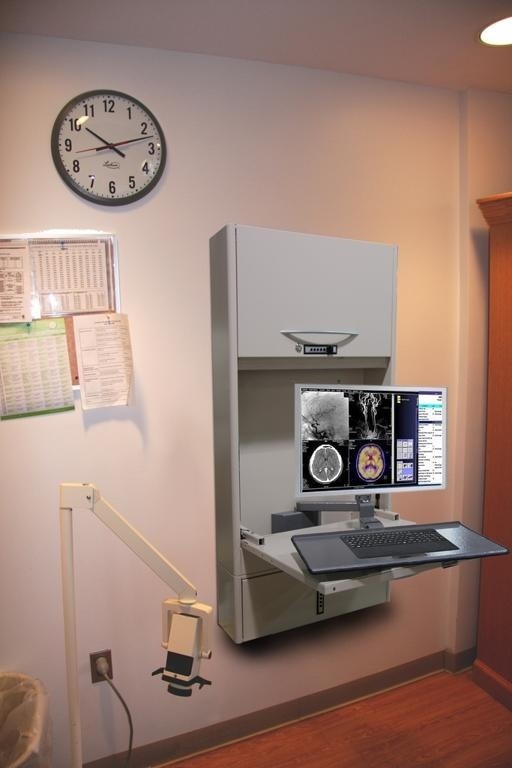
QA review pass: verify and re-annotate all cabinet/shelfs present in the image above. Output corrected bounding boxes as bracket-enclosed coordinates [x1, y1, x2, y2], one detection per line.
[207, 223, 397, 647]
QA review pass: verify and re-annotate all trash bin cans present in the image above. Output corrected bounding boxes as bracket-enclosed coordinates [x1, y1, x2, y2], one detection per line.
[0, 672, 45, 768]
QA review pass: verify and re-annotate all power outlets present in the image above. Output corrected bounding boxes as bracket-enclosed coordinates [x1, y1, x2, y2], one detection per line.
[90, 648, 113, 683]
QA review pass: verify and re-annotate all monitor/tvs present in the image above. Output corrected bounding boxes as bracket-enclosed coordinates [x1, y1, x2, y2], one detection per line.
[294, 383, 448, 498]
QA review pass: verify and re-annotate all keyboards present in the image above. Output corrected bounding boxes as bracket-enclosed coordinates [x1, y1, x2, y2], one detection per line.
[340, 528, 460, 560]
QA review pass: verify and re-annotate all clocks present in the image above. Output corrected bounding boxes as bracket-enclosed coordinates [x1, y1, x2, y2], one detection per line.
[49, 87, 166, 207]
